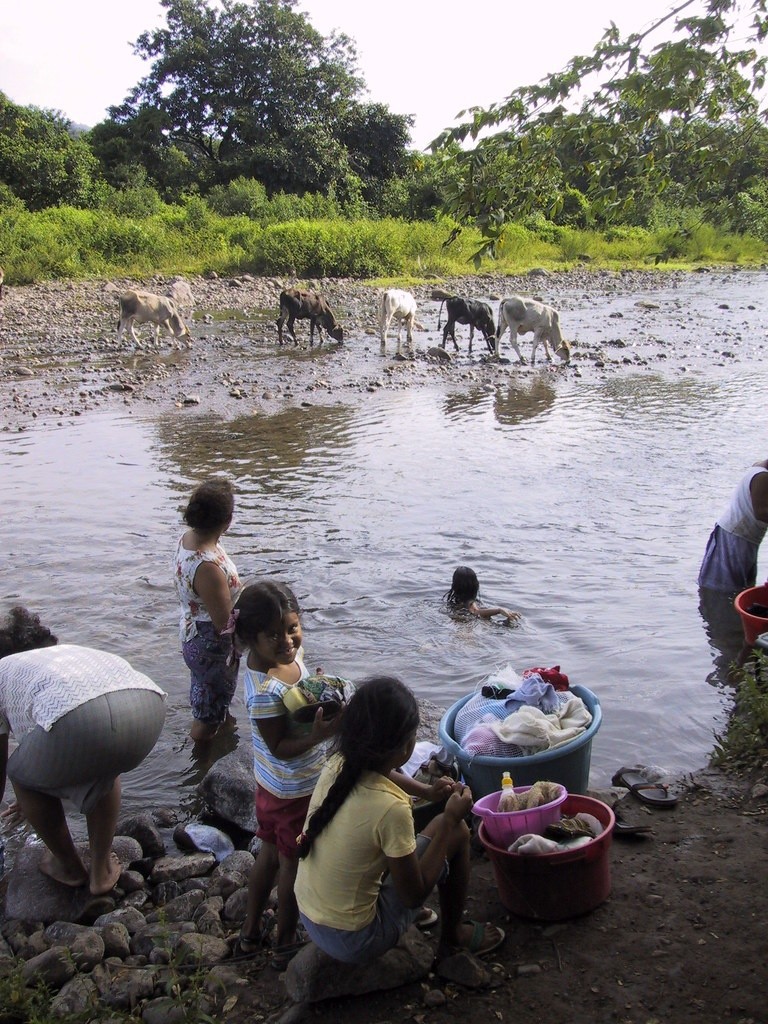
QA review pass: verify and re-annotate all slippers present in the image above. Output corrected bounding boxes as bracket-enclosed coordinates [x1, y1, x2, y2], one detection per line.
[620, 772, 679, 809]
[453, 919, 506, 956]
[605, 802, 654, 834]
[411, 907, 440, 929]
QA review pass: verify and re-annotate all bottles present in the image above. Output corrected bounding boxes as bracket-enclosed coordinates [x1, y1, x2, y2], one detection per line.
[500, 771, 516, 800]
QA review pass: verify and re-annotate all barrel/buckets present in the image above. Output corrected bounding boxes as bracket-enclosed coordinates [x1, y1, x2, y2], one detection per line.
[478, 794, 615, 922]
[471, 782, 568, 849]
[437, 683, 601, 795]
[734, 585, 768, 645]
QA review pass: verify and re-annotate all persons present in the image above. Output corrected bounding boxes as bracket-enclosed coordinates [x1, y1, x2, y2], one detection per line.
[295, 676, 507, 956]
[0, 608, 166, 895]
[229, 579, 343, 970]
[454, 566, 521, 620]
[175, 478, 244, 738]
[697, 460, 768, 594]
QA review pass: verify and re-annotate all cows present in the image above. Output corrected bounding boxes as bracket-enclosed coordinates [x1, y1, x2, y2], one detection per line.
[437, 295, 497, 355]
[484, 295, 571, 367]
[379, 289, 425, 342]
[276, 287, 349, 346]
[115, 288, 195, 351]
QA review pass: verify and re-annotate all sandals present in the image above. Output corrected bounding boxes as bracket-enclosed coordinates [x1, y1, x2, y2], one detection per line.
[268, 928, 313, 970]
[234, 908, 275, 955]
